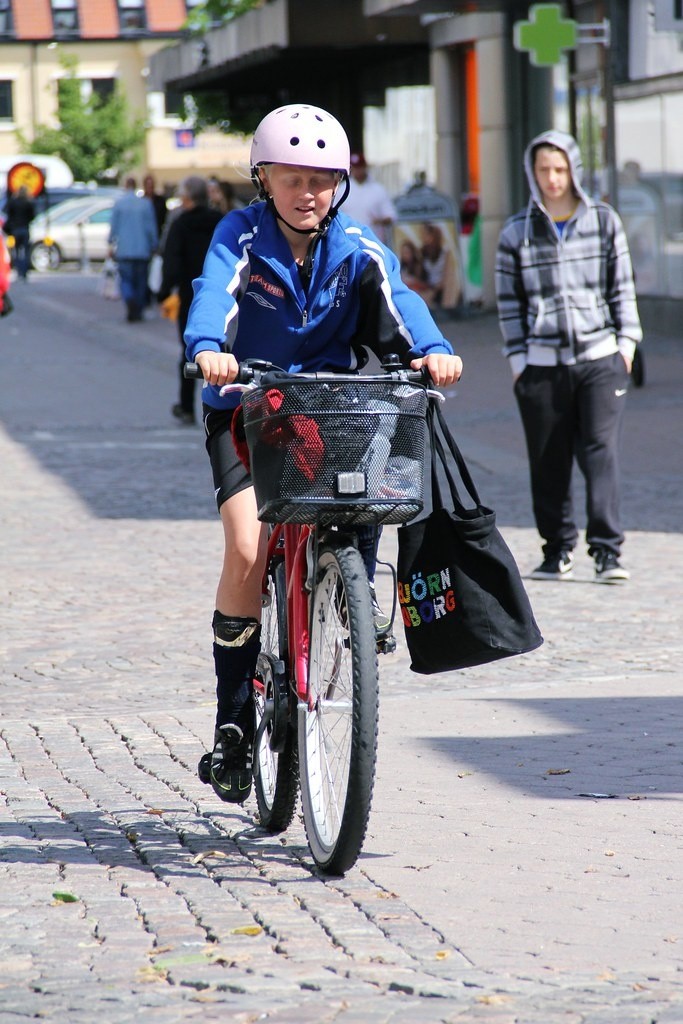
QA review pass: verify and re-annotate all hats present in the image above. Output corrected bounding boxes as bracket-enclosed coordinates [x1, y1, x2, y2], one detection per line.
[350, 153, 365, 167]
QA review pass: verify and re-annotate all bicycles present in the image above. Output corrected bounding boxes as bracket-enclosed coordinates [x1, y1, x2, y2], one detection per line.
[183, 352, 462, 876]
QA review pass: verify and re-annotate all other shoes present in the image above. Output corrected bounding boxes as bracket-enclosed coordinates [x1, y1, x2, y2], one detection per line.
[127, 299, 143, 320]
[173, 405, 196, 425]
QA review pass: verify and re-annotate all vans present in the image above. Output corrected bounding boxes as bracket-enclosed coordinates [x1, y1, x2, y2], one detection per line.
[0, 187, 125, 271]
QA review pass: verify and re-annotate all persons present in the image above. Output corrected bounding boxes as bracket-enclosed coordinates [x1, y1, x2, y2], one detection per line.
[400, 221, 460, 309]
[2, 186, 37, 279]
[105, 175, 236, 421]
[495, 132, 642, 580]
[332, 156, 396, 252]
[617, 162, 669, 290]
[183, 104, 463, 803]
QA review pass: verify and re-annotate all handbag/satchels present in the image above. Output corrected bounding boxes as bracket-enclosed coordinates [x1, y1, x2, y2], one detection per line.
[147, 254, 163, 294]
[397, 363, 544, 675]
[96, 257, 121, 301]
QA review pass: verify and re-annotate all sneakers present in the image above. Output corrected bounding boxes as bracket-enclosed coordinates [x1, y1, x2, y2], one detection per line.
[335, 578, 393, 638]
[202, 696, 257, 803]
[531, 550, 574, 579]
[593, 550, 629, 579]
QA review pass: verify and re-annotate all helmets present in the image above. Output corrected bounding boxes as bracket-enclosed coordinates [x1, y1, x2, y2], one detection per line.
[249, 104, 350, 177]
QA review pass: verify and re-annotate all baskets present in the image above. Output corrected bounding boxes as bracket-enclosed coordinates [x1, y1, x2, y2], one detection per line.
[239, 378, 429, 526]
